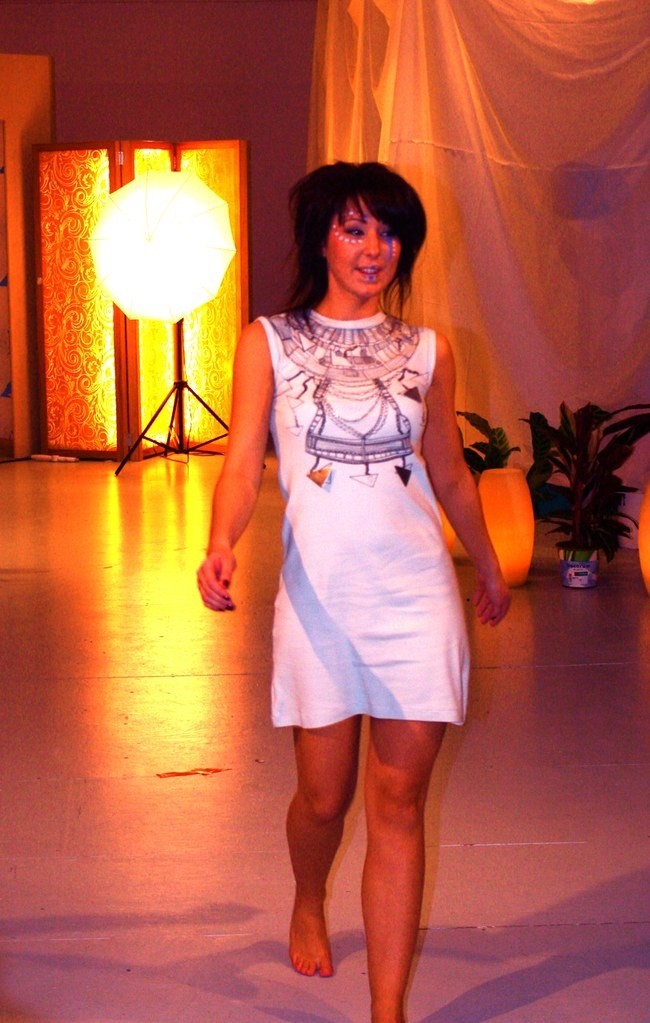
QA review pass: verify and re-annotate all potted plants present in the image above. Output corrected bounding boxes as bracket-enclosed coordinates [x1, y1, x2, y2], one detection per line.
[456, 400, 650, 589]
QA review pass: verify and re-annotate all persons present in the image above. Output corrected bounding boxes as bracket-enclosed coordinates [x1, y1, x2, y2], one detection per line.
[196, 161, 512, 1023]
